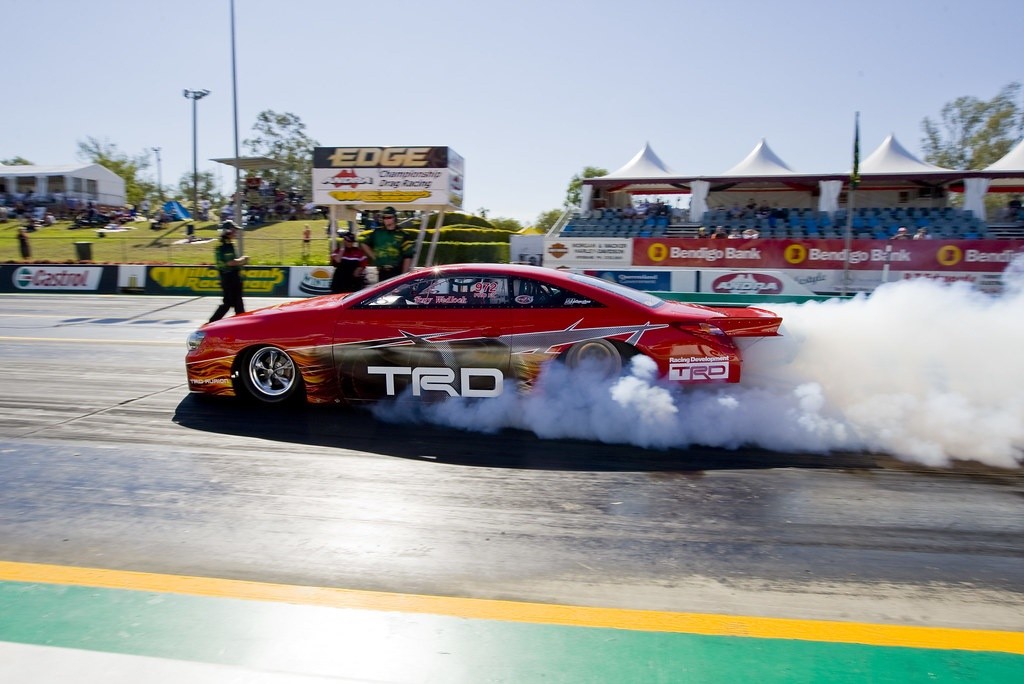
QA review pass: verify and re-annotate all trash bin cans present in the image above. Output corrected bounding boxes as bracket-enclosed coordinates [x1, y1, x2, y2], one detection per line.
[186, 225, 194, 235]
[73, 243, 93, 260]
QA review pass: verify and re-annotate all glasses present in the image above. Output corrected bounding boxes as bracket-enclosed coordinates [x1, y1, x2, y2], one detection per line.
[381, 216, 394, 219]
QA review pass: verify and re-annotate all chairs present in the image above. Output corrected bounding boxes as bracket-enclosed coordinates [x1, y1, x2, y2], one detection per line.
[558, 208, 999, 240]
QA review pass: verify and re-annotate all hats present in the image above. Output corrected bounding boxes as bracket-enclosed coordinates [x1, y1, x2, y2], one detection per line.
[375, 207, 395, 215]
[221, 220, 244, 230]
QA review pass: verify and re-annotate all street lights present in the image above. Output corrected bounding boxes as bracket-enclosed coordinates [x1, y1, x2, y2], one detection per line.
[152, 145, 163, 198]
[184, 88, 211, 222]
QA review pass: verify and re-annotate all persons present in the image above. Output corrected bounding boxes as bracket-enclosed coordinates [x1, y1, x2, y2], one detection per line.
[16, 227, 32, 260]
[302, 225, 312, 256]
[624, 199, 768, 239]
[210, 220, 250, 323]
[330, 232, 368, 295]
[360, 206, 417, 283]
[16, 196, 314, 231]
[1002, 195, 1021, 221]
[890, 226, 932, 241]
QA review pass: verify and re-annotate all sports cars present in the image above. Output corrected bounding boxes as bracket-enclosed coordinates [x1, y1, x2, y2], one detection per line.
[184, 263, 789, 419]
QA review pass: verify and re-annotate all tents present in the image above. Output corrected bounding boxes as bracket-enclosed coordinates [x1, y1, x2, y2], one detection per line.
[579, 133, 1024, 222]
